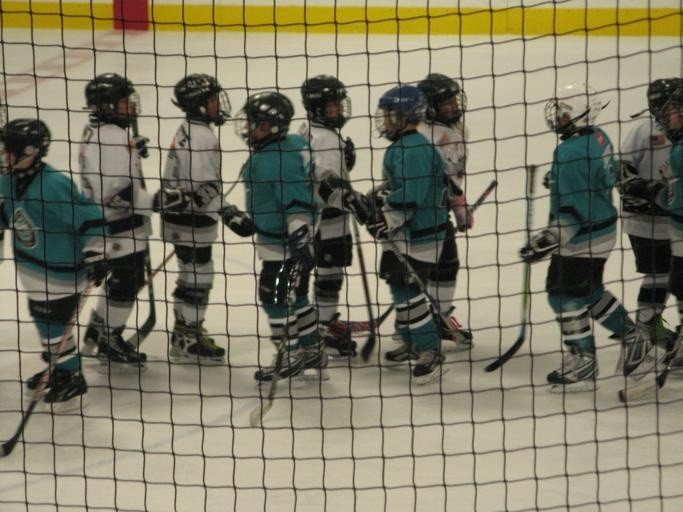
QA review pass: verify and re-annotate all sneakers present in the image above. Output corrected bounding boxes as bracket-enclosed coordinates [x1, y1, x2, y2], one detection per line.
[100, 318, 148, 362]
[170, 317, 225, 358]
[609, 318, 654, 377]
[252, 337, 305, 381]
[26, 365, 88, 402]
[84, 308, 104, 347]
[384, 339, 414, 361]
[429, 304, 473, 344]
[322, 334, 358, 352]
[300, 347, 328, 369]
[411, 349, 447, 378]
[545, 337, 600, 384]
[634, 309, 674, 348]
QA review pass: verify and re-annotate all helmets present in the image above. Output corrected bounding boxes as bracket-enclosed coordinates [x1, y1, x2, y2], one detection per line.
[372, 83, 424, 141]
[647, 78, 671, 118]
[170, 72, 232, 127]
[544, 82, 600, 135]
[234, 91, 296, 150]
[662, 83, 683, 143]
[0, 117, 52, 174]
[299, 73, 351, 130]
[417, 73, 467, 124]
[81, 71, 140, 128]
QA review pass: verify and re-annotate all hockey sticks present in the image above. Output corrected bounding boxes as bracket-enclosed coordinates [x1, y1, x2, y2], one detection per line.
[382, 234, 472, 344]
[339, 180, 497, 330]
[124, 117, 155, 351]
[618, 314, 683, 401]
[353, 217, 375, 364]
[1, 281, 93, 458]
[484, 164, 536, 373]
[251, 253, 297, 429]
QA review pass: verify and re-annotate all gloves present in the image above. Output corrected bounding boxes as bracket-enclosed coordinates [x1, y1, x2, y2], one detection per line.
[519, 229, 559, 263]
[365, 209, 391, 241]
[85, 252, 111, 287]
[221, 206, 255, 237]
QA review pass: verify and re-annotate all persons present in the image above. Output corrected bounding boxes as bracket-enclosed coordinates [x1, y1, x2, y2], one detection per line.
[648, 86, 683, 367]
[80, 74, 193, 376]
[394, 74, 473, 348]
[234, 91, 330, 389]
[299, 74, 368, 357]
[161, 74, 256, 365]
[520, 82, 659, 395]
[616, 80, 680, 353]
[0, 117, 109, 414]
[367, 83, 450, 385]
